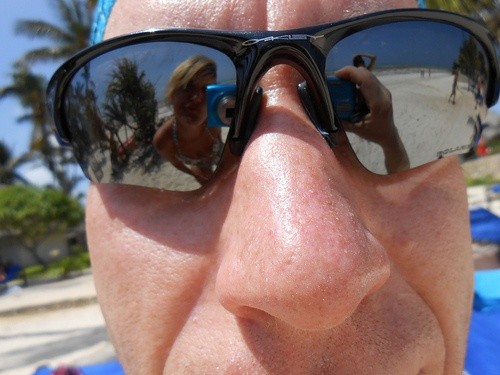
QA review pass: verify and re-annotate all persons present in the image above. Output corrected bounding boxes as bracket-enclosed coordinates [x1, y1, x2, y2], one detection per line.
[151, 54, 226, 189]
[447, 68, 459, 105]
[354, 53, 379, 71]
[331, 64, 411, 175]
[17, 1, 494, 375]
[467, 61, 484, 109]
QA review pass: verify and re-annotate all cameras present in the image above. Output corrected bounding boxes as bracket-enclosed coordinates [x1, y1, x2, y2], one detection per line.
[206, 77, 357, 126]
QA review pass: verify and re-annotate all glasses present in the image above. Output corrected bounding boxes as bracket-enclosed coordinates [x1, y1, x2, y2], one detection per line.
[42, 13, 500, 192]
